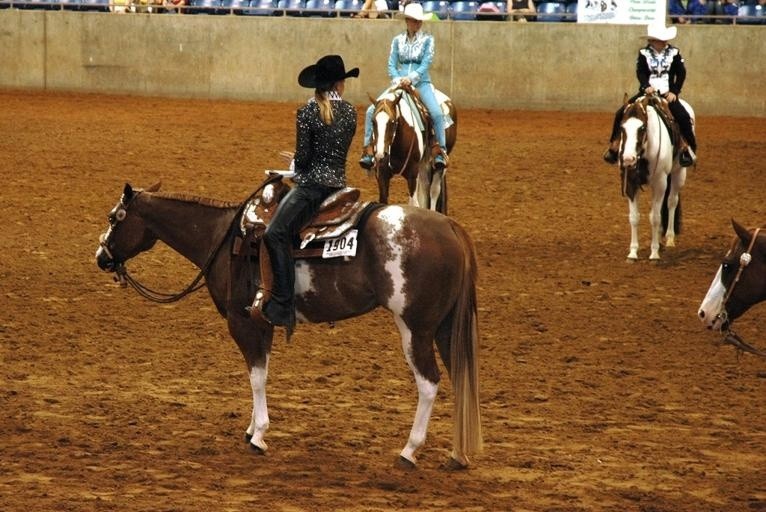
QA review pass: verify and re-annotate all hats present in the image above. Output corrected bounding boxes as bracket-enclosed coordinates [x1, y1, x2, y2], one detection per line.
[396, 2, 433, 21]
[297, 54, 359, 88]
[638, 21, 677, 42]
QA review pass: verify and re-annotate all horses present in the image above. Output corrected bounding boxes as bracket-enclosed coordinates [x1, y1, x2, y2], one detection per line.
[366, 87, 458, 218]
[619, 92, 697, 264]
[697, 217, 766, 332]
[94, 180, 483, 471]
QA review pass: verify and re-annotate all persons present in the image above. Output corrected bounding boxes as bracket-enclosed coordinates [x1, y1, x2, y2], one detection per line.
[245, 54, 361, 324]
[359, 5, 450, 172]
[100, 0, 766, 24]
[604, 20, 698, 168]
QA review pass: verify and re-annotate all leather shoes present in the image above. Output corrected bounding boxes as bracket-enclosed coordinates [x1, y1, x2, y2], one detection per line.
[603, 149, 618, 163]
[434, 155, 446, 170]
[678, 149, 693, 167]
[358, 156, 374, 168]
[244, 307, 282, 327]
